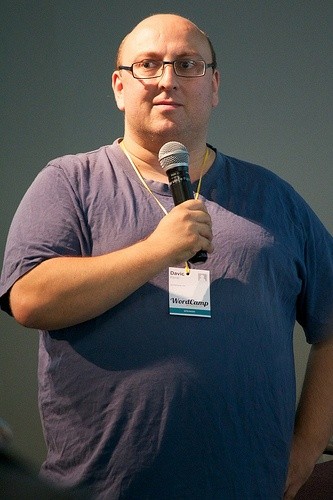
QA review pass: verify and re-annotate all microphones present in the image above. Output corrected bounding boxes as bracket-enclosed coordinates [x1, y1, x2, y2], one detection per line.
[158, 141, 207, 264]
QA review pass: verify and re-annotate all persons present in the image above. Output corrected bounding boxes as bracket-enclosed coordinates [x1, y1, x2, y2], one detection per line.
[0, 13, 333, 500]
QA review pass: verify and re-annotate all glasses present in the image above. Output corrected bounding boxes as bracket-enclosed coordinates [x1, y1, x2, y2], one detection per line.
[118, 58, 216, 80]
